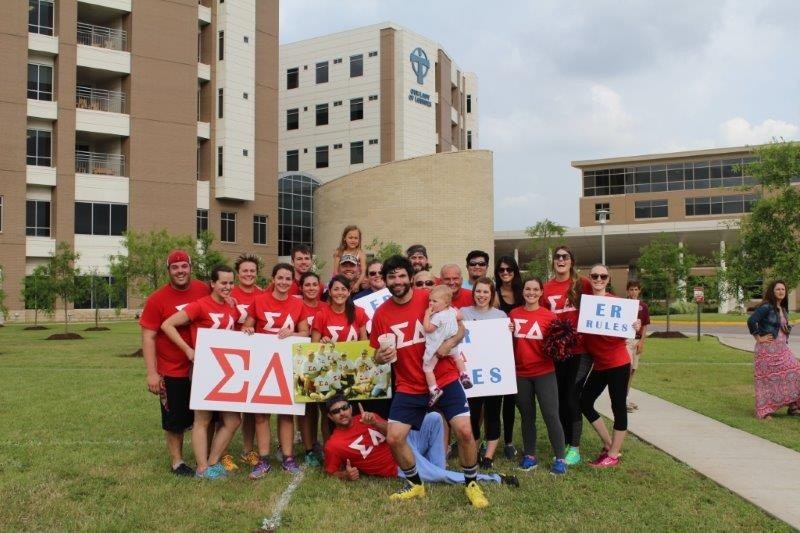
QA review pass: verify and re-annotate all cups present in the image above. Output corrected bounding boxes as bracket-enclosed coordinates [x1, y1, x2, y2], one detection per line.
[378, 333, 399, 363]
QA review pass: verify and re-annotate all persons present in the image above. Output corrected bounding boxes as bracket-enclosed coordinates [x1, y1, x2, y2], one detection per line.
[367, 254, 491, 509]
[141, 223, 650, 510]
[745, 280, 800, 422]
[421, 286, 473, 406]
[323, 396, 520, 490]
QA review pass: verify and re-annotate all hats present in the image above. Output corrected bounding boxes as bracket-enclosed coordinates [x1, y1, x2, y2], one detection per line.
[340, 254, 357, 265]
[166, 251, 189, 268]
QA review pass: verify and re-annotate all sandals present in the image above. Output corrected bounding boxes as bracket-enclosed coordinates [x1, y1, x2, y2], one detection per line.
[788, 407, 800, 416]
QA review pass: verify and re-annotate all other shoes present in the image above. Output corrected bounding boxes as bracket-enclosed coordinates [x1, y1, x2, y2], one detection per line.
[460, 373, 474, 389]
[429, 387, 443, 408]
[626, 402, 638, 412]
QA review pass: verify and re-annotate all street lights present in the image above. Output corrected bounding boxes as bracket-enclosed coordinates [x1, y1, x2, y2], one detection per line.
[595, 206, 616, 266]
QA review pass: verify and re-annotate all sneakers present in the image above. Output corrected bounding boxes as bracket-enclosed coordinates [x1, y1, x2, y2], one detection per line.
[599, 447, 622, 460]
[500, 473, 520, 487]
[172, 463, 194, 477]
[305, 451, 319, 467]
[196, 468, 218, 480]
[589, 454, 620, 467]
[292, 431, 303, 443]
[464, 481, 489, 508]
[552, 458, 566, 472]
[552, 444, 569, 461]
[249, 457, 271, 479]
[312, 441, 324, 460]
[390, 483, 425, 500]
[211, 462, 226, 475]
[481, 457, 493, 470]
[504, 444, 517, 458]
[564, 447, 581, 465]
[282, 455, 300, 473]
[276, 448, 283, 461]
[241, 452, 258, 464]
[518, 455, 537, 470]
[221, 454, 234, 470]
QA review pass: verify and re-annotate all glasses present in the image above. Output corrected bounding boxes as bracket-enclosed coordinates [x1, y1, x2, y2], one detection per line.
[330, 403, 350, 414]
[369, 270, 383, 276]
[498, 267, 514, 272]
[554, 254, 570, 260]
[414, 280, 435, 286]
[469, 261, 487, 266]
[590, 273, 609, 280]
